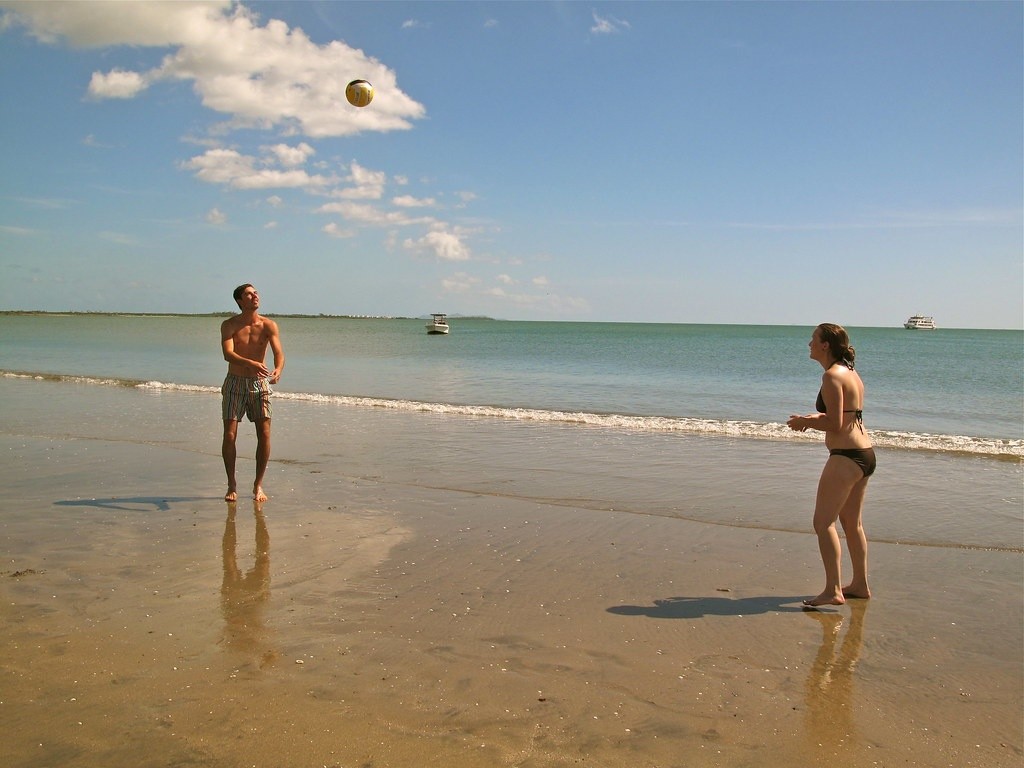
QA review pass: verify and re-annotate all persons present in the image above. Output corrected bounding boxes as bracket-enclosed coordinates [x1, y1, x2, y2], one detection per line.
[787, 323, 876, 606]
[221, 505, 279, 671]
[221, 284, 285, 501]
[803, 599, 867, 744]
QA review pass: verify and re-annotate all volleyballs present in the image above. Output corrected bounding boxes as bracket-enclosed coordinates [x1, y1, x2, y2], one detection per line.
[346, 79, 374, 107]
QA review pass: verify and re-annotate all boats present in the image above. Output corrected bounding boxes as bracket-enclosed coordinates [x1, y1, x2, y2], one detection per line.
[903, 314, 935, 330]
[425, 313, 449, 334]
